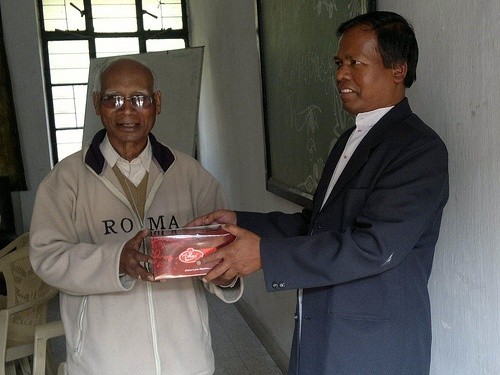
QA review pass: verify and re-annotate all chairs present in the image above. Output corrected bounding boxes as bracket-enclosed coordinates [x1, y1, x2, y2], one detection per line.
[0, 231, 59, 375]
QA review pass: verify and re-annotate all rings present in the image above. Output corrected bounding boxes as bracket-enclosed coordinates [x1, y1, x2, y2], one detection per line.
[137, 274, 142, 280]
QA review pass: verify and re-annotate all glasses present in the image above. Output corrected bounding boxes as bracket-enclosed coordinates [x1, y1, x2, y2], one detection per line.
[100, 94, 154, 109]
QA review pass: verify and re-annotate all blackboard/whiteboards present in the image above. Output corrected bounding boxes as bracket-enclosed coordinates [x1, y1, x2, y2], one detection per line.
[255, 0, 369, 208]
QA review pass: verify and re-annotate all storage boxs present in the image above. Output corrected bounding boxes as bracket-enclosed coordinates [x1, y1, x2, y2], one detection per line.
[144, 224, 237, 280]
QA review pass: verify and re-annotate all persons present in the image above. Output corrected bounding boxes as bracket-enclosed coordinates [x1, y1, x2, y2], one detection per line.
[30, 60, 245, 374]
[182, 11, 451, 375]
